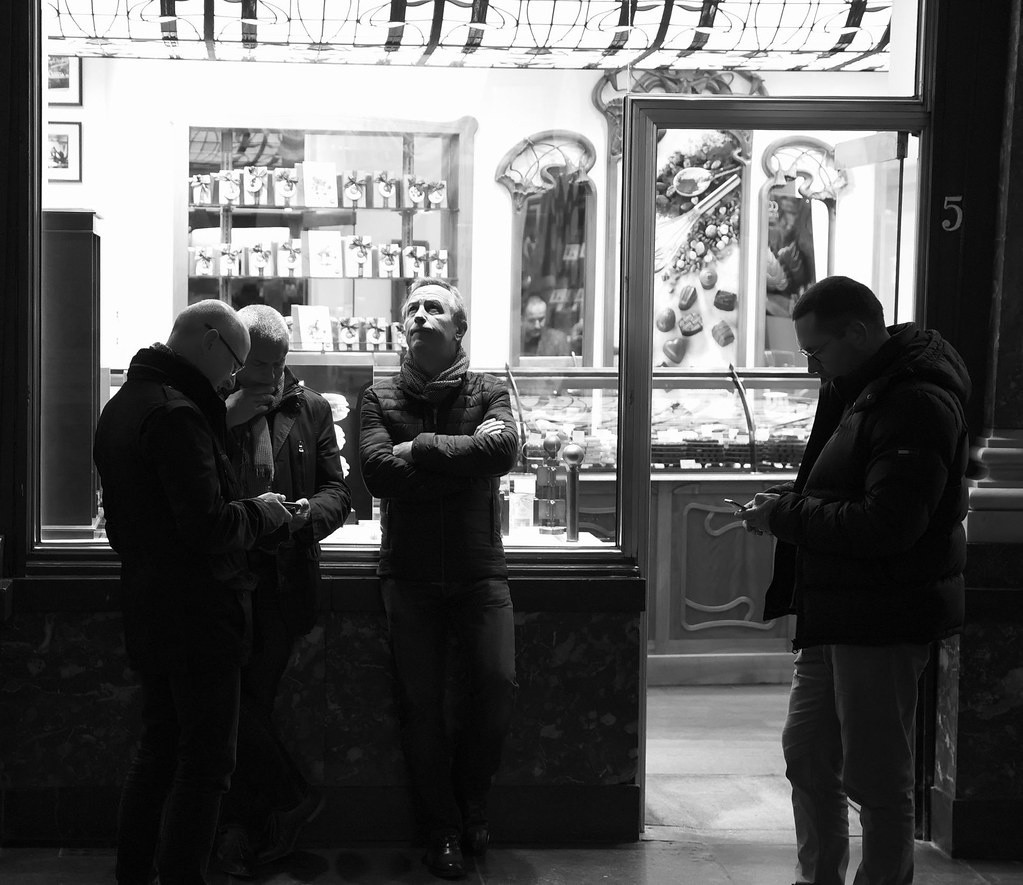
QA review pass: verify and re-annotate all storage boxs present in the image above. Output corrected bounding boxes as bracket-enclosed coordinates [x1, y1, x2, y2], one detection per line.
[365, 316, 387, 351]
[338, 317, 359, 351]
[371, 171, 397, 208]
[342, 169, 367, 208]
[248, 243, 273, 277]
[277, 238, 303, 278]
[428, 180, 448, 209]
[428, 249, 448, 278]
[399, 174, 424, 208]
[271, 168, 298, 206]
[192, 175, 211, 204]
[218, 170, 240, 205]
[218, 243, 239, 276]
[243, 166, 268, 205]
[345, 235, 373, 278]
[401, 246, 427, 278]
[377, 243, 401, 278]
[391, 322, 409, 351]
[194, 246, 214, 276]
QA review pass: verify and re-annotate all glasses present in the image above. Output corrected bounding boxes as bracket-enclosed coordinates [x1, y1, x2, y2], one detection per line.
[205, 323, 247, 376]
[801, 326, 843, 358]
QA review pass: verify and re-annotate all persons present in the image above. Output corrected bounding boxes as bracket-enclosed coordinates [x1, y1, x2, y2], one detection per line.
[93, 298, 351, 885]
[734, 276, 971, 885]
[520, 294, 572, 357]
[359, 277, 519, 879]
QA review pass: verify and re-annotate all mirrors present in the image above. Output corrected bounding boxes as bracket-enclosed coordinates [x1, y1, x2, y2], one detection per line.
[494, 128, 598, 398]
[754, 136, 836, 367]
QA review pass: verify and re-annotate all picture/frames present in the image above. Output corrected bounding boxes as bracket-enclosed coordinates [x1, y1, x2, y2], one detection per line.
[48, 56, 82, 107]
[47, 121, 82, 183]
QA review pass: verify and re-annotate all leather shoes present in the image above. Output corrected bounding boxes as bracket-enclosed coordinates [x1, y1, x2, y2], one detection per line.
[426, 828, 467, 879]
[251, 790, 323, 868]
[463, 824, 490, 858]
[214, 820, 253, 878]
